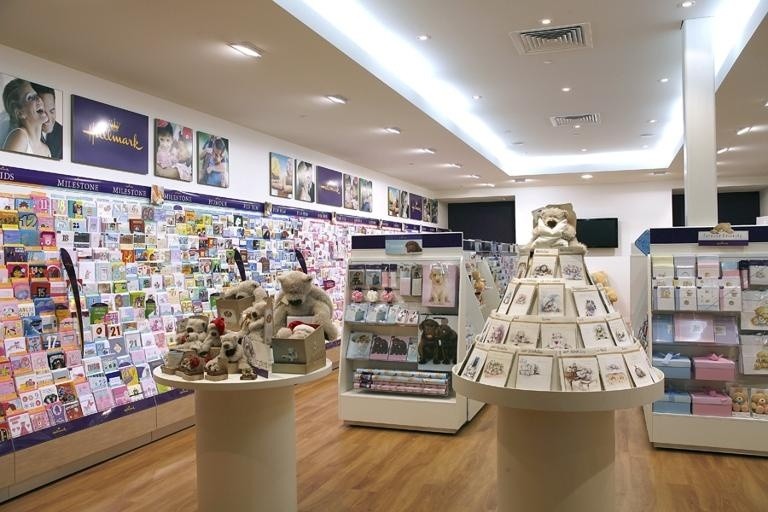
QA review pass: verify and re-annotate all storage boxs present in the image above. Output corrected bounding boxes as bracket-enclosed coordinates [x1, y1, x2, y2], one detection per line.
[652, 355, 735, 417]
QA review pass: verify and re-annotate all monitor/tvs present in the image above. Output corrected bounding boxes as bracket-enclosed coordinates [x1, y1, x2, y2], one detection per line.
[577, 217, 618, 248]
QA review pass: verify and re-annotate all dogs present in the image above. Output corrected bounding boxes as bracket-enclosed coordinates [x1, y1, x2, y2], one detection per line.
[427, 265, 453, 305]
[418, 319, 444, 365]
[435, 324, 458, 365]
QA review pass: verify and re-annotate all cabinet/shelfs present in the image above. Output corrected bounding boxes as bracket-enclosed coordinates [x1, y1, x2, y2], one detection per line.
[629, 223, 768, 458]
[337, 229, 517, 436]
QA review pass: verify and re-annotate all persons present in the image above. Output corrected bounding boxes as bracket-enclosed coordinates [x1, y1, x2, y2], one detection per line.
[269, 151, 439, 223]
[153, 118, 231, 189]
[2, 77, 52, 158]
[29, 82, 63, 160]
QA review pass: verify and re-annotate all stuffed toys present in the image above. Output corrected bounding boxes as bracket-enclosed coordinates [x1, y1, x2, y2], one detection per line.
[529, 206, 577, 252]
[174, 268, 339, 376]
[729, 386, 750, 413]
[750, 391, 768, 414]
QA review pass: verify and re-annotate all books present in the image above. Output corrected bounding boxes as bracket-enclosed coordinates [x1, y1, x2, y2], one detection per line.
[456, 239, 660, 392]
[166, 208, 281, 330]
[282, 214, 403, 337]
[0, 189, 176, 444]
